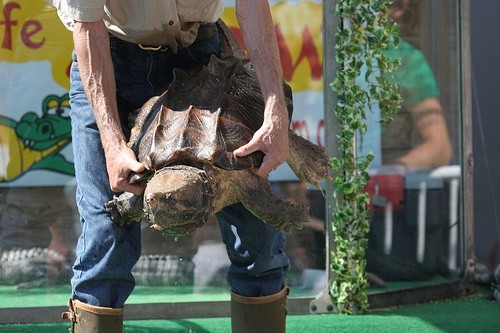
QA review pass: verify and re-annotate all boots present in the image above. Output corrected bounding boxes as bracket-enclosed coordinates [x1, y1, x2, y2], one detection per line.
[231, 284, 288, 333]
[61, 298, 123, 333]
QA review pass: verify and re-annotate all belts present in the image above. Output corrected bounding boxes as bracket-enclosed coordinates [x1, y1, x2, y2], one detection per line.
[138, 42, 165, 51]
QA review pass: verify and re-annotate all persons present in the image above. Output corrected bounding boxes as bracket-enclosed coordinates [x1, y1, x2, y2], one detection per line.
[51, 0, 296, 333]
[373, 1, 455, 168]
